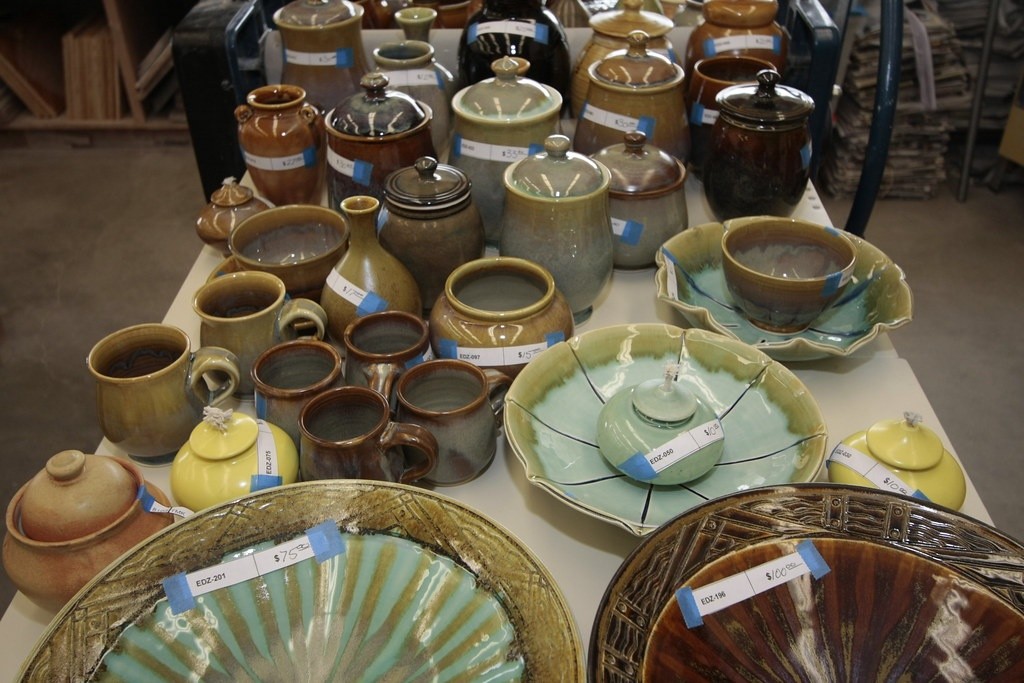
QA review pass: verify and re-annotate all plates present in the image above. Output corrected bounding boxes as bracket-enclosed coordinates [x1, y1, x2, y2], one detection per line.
[588, 483, 1024, 683]
[502, 322, 829, 535]
[205, 254, 240, 286]
[655, 215, 913, 363]
[13, 479, 588, 683]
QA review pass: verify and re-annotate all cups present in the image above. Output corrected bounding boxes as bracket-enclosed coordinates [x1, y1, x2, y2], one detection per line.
[688, 54, 778, 182]
[86, 270, 329, 459]
[251, 310, 496, 487]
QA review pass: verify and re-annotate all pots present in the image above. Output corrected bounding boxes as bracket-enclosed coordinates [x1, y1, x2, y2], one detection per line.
[229, 204, 349, 292]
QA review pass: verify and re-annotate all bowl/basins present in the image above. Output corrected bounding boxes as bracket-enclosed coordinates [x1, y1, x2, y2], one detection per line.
[720, 218, 859, 335]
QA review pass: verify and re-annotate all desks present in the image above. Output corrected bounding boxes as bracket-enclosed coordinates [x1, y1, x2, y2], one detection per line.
[1, 28, 997, 683]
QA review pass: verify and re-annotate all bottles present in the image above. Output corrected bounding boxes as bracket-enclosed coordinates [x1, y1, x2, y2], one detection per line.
[393, 7, 438, 41]
[372, 40, 457, 157]
[320, 195, 423, 358]
[234, 84, 327, 209]
[428, 256, 575, 379]
